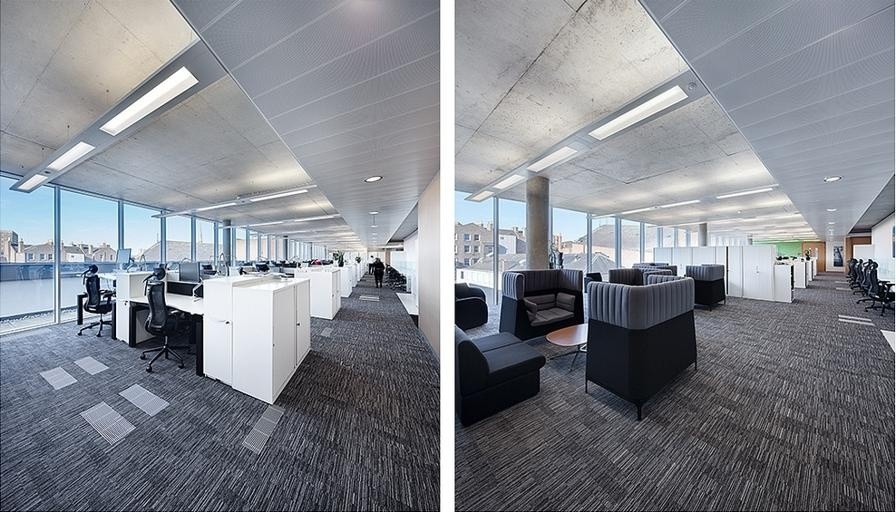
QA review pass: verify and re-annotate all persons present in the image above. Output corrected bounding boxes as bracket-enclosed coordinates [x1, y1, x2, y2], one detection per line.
[372, 258, 385, 288]
[367, 256, 375, 275]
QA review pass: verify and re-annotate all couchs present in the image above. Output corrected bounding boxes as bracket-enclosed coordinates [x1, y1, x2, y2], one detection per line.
[496, 263, 585, 346]
[577, 272, 700, 423]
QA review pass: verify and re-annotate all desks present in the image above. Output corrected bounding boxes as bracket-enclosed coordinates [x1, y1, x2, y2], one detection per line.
[70, 255, 404, 407]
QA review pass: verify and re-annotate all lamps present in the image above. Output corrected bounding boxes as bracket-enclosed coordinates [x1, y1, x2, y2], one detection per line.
[7, 36, 229, 193]
[460, 67, 712, 203]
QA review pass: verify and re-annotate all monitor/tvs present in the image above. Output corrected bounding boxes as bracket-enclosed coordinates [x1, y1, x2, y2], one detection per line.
[179, 262, 200, 283]
[116, 249, 131, 272]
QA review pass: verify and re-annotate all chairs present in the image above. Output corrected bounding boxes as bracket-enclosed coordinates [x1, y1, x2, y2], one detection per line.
[844, 253, 895, 319]
[453, 321, 548, 430]
[453, 281, 489, 331]
[582, 260, 729, 318]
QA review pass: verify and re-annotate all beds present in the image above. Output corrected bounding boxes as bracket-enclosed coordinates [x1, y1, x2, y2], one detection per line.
[543, 322, 589, 379]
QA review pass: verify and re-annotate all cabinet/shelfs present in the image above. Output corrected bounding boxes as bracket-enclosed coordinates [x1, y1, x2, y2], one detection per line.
[771, 255, 818, 304]
